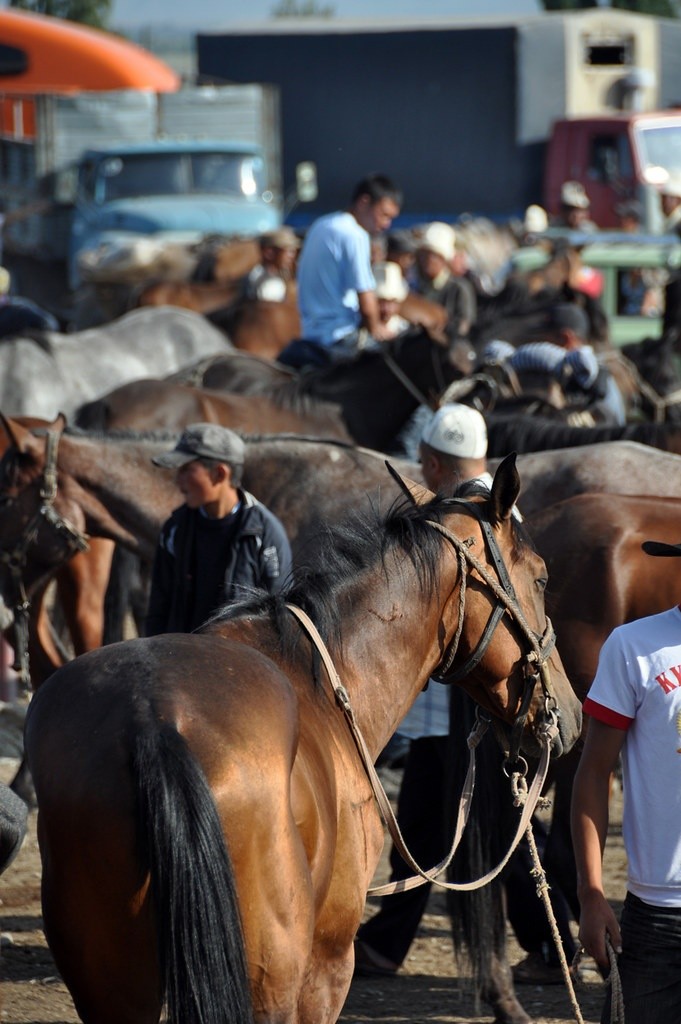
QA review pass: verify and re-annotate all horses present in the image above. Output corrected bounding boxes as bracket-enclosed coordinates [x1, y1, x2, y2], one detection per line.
[0, 229, 680, 1024]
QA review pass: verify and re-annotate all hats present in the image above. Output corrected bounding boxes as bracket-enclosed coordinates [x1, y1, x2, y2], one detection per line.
[474, 339, 516, 368]
[150, 422, 246, 470]
[658, 177, 681, 198]
[258, 227, 302, 250]
[565, 266, 602, 298]
[561, 181, 590, 209]
[525, 205, 549, 232]
[422, 403, 487, 459]
[411, 219, 455, 260]
[504, 342, 568, 373]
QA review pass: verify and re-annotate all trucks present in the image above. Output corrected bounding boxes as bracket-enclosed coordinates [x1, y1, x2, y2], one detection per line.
[0, 81, 286, 351]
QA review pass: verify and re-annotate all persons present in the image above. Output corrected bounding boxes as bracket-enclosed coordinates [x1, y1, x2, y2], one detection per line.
[571, 541, 681, 1024]
[355, 404, 585, 987]
[145, 423, 293, 635]
[295, 173, 406, 360]
[249, 179, 681, 426]
